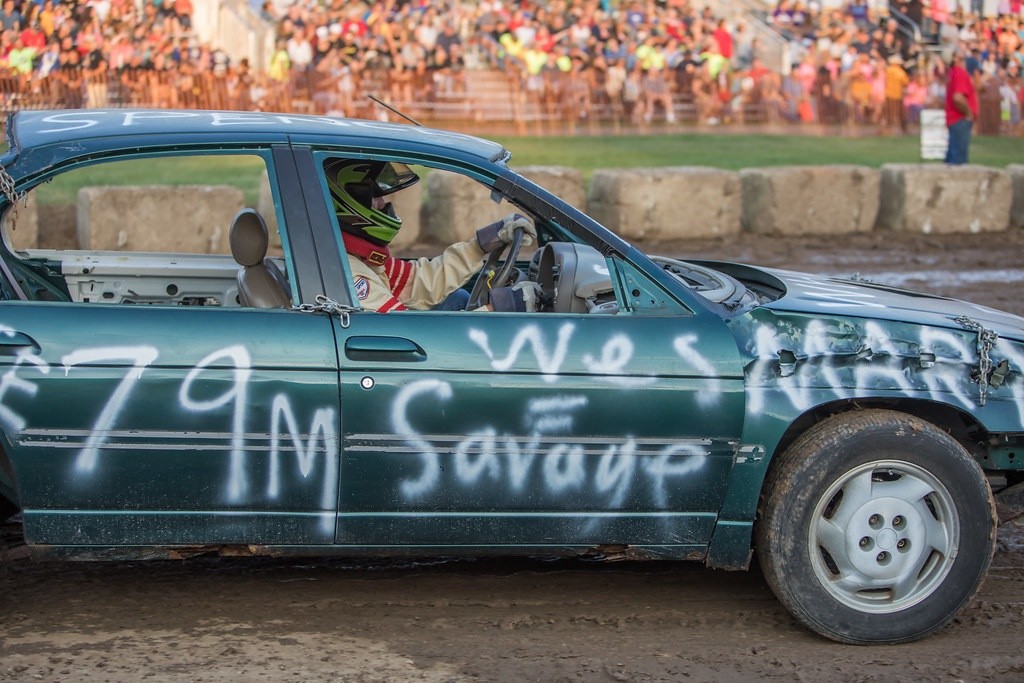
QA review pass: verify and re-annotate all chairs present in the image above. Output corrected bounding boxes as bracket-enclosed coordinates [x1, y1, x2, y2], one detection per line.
[229, 208, 291, 310]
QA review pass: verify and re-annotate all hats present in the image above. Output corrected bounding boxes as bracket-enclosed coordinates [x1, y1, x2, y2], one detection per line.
[940, 44, 955, 66]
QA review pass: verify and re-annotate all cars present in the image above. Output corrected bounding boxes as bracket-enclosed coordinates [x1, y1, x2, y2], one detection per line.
[2, 110, 1023, 646]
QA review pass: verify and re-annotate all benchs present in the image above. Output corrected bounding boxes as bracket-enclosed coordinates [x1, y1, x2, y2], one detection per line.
[407, 66, 772, 123]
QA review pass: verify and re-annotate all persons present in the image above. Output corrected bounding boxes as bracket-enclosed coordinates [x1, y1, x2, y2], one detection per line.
[0, 0, 1024, 129]
[942, 44, 978, 163]
[326, 158, 551, 312]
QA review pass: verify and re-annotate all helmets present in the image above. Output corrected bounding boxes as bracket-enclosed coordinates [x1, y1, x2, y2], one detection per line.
[323, 157, 420, 247]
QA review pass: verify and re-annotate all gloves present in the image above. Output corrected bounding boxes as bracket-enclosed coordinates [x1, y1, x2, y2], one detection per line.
[487, 281, 545, 312]
[476, 214, 536, 253]
